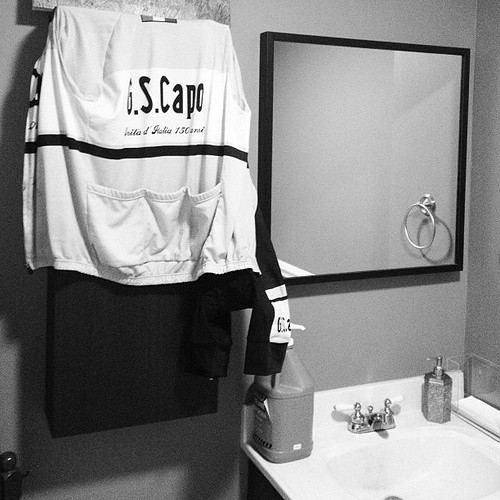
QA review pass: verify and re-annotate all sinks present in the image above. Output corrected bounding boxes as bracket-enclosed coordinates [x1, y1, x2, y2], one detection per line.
[241, 370, 500, 500]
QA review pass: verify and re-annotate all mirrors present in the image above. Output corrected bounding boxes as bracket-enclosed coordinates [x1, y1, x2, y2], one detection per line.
[259, 31, 472, 285]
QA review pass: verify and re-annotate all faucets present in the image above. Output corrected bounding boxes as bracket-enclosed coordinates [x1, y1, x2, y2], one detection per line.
[334, 395, 405, 434]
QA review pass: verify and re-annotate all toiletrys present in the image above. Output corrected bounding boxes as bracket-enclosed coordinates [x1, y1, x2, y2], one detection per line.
[252, 321, 316, 465]
[421, 356, 453, 425]
